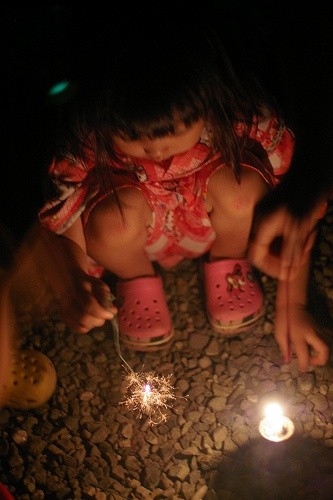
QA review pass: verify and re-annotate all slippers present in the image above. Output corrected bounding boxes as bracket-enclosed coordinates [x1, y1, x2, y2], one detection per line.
[116, 276, 175, 352]
[204, 257, 266, 335]
[1, 351, 57, 410]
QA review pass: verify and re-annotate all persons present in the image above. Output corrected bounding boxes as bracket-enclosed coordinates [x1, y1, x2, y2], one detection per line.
[0, 269, 57, 410]
[248, 170, 333, 281]
[38, 41, 333, 368]
